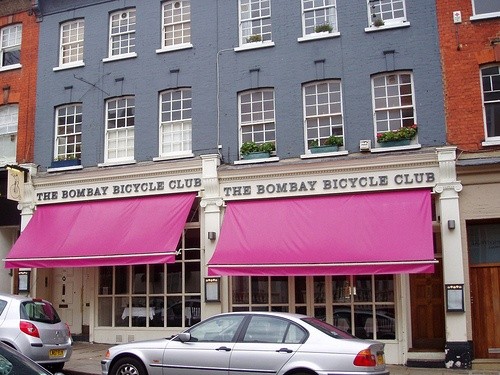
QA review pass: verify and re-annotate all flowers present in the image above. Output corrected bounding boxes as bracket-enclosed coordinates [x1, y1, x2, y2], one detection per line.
[377, 124, 417, 142]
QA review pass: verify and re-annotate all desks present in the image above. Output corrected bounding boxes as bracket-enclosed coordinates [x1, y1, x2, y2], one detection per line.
[122, 306, 154, 327]
[186, 299, 201, 327]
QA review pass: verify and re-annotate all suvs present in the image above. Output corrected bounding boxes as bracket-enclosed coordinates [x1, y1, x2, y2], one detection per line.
[0, 292, 74, 374]
[155, 299, 201, 322]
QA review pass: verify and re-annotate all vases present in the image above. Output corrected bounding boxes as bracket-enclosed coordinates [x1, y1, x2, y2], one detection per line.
[378, 139, 411, 147]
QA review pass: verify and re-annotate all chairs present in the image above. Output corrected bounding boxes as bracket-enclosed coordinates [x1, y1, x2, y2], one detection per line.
[190, 302, 202, 326]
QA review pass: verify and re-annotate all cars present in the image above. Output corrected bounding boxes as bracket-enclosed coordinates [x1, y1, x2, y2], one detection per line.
[316, 308, 395, 340]
[100, 311, 390, 375]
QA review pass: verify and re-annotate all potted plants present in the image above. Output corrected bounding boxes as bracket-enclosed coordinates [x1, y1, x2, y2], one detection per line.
[51, 154, 81, 168]
[241, 140, 275, 159]
[311, 135, 343, 154]
